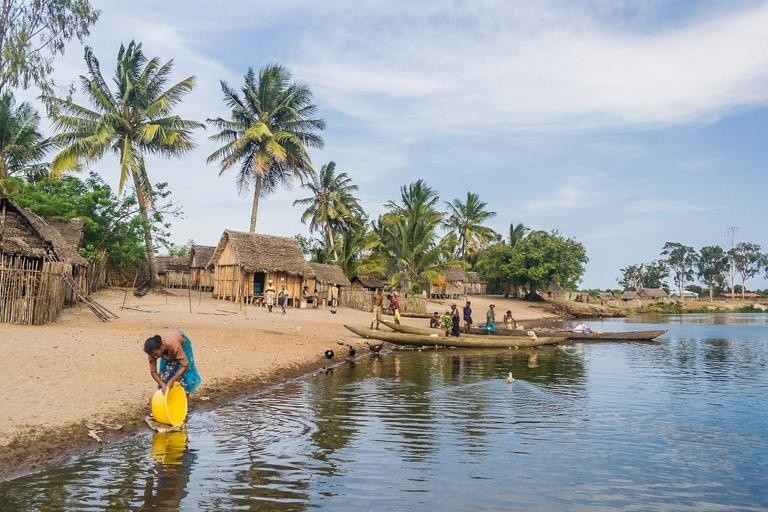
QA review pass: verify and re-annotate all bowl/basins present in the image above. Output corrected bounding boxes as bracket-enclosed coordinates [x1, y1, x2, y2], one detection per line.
[151, 383, 187, 427]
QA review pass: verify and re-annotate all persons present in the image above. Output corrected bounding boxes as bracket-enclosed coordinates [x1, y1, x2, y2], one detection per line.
[303, 283, 319, 309]
[485, 302, 497, 335]
[276, 284, 289, 315]
[369, 288, 385, 330]
[143, 326, 202, 399]
[387, 294, 401, 332]
[503, 310, 517, 331]
[265, 280, 276, 312]
[429, 301, 473, 337]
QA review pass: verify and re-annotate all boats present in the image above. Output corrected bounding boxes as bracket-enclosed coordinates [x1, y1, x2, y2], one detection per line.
[377, 317, 569, 344]
[343, 324, 556, 348]
[455, 324, 667, 340]
